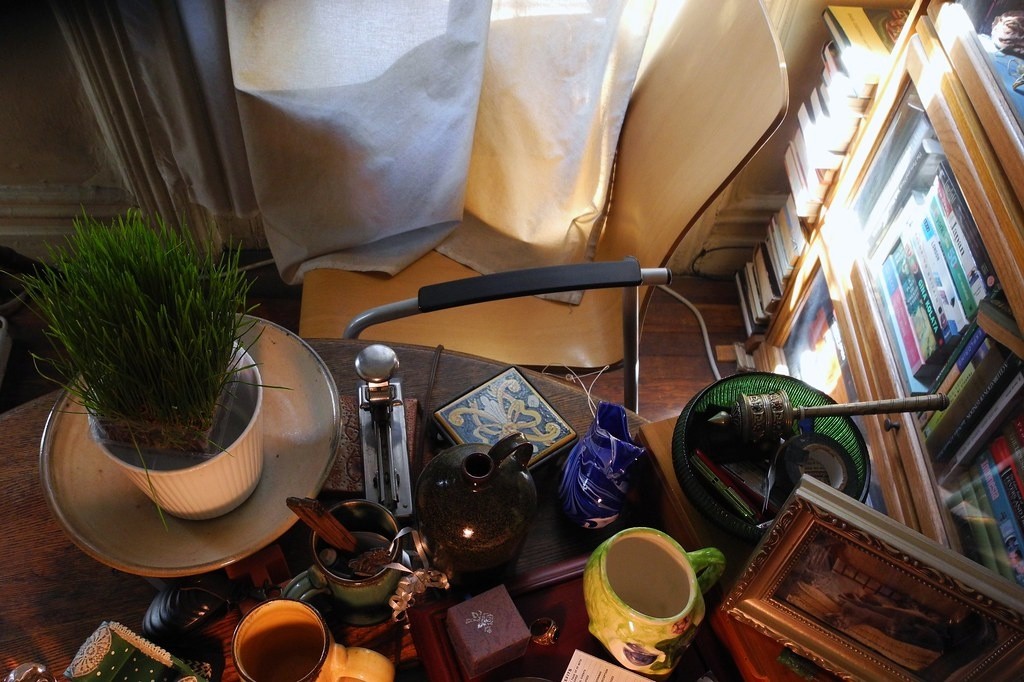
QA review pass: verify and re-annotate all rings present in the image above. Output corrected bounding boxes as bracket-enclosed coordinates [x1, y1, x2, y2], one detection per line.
[530, 617, 561, 646]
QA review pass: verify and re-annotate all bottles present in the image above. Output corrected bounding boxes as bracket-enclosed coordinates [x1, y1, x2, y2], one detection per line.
[413, 432, 536, 598]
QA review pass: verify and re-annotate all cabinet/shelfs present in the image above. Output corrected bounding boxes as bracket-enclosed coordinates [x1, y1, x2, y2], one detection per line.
[723, 0, 1024, 682]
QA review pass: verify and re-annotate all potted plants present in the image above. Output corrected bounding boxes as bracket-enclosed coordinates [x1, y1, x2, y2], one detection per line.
[0, 202, 292, 532]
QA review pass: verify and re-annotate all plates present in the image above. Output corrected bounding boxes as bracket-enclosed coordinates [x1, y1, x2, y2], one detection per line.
[36, 311, 341, 578]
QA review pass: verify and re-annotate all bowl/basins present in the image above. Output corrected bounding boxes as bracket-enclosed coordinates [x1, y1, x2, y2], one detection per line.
[670, 370, 872, 541]
[0, 267, 29, 317]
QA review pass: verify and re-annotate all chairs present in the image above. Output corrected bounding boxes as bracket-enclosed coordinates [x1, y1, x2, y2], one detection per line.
[297, 0, 791, 413]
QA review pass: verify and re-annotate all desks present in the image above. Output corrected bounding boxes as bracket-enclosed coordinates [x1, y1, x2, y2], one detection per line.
[0, 335, 745, 682]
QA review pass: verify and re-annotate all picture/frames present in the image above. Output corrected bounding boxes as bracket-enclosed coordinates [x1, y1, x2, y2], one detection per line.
[725, 475, 1024, 682]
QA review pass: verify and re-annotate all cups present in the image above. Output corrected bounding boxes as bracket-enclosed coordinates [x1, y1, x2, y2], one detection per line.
[583, 527, 727, 681]
[280, 498, 403, 627]
[230, 596, 396, 682]
[3, 662, 57, 682]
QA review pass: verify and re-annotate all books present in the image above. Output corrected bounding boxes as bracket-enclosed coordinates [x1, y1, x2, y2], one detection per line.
[735, 5, 1024, 588]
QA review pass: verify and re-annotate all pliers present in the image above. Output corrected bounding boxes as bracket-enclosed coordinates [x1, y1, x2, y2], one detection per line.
[760, 462, 775, 514]
